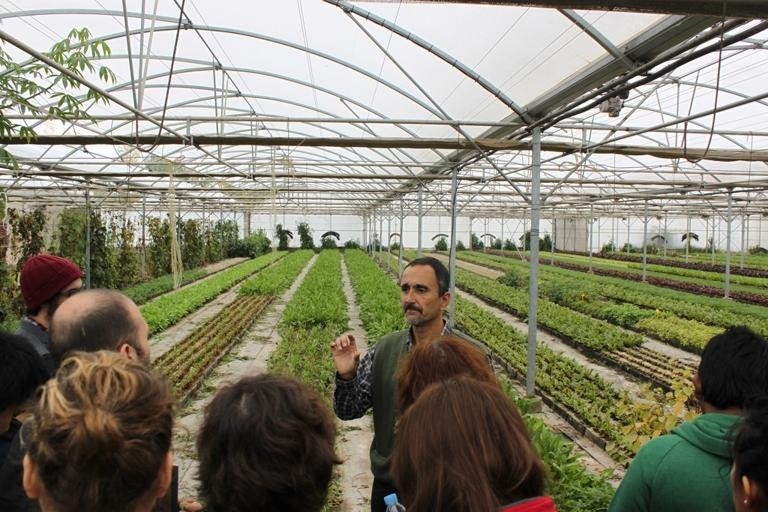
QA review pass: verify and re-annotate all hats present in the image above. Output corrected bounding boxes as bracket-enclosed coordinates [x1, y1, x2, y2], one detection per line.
[20, 254, 82, 309]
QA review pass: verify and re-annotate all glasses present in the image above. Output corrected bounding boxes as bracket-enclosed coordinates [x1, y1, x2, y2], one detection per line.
[58, 290, 79, 298]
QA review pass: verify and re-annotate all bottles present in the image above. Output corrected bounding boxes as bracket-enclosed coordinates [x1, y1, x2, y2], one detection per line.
[383, 493, 406, 512]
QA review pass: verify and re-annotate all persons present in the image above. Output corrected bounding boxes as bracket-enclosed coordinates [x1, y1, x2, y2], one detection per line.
[392, 335, 503, 419]
[22, 348, 180, 511]
[385, 375, 558, 512]
[0, 328, 49, 473]
[1, 287, 202, 511]
[183, 373, 348, 512]
[713, 406, 768, 512]
[12, 252, 85, 358]
[329, 256, 496, 511]
[606, 324, 767, 512]
